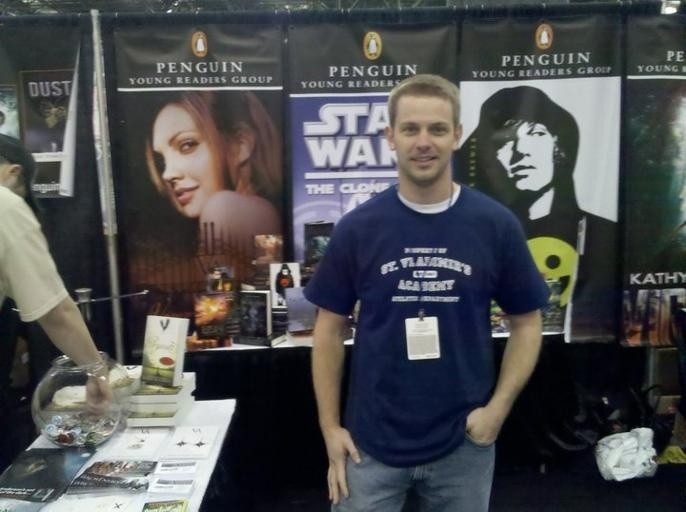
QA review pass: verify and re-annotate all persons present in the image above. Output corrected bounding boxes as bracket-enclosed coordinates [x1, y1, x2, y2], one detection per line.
[0, 133, 113, 418]
[301, 75, 552, 512]
[457, 85, 618, 343]
[144, 91, 283, 290]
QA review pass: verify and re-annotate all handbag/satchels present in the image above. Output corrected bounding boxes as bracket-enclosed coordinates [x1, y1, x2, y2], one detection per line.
[626, 384, 675, 453]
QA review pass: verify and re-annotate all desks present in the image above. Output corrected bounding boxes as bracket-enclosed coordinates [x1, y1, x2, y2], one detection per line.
[1, 369, 236, 511]
[157, 310, 566, 512]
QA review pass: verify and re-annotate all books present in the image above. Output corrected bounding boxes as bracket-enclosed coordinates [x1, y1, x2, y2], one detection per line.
[240, 290, 273, 336]
[194, 292, 231, 347]
[233, 331, 287, 346]
[141, 315, 189, 386]
[0, 364, 221, 512]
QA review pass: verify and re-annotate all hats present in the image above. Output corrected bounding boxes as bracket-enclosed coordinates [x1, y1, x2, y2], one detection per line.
[0, 133, 44, 214]
[457, 86, 579, 190]
